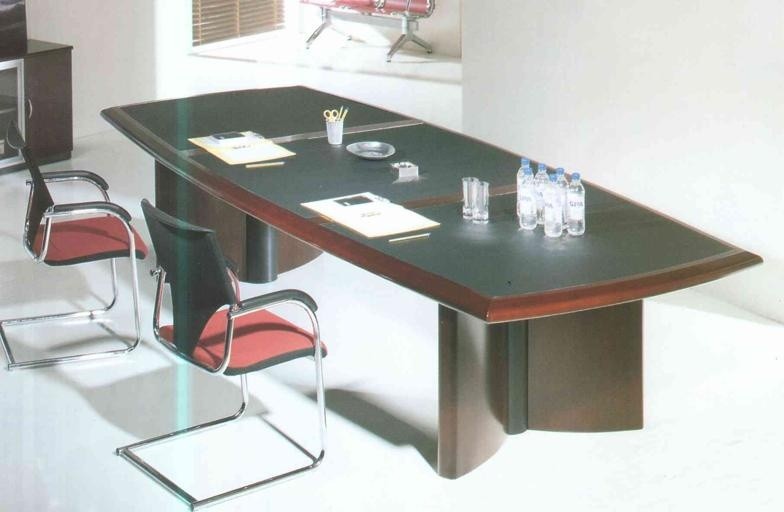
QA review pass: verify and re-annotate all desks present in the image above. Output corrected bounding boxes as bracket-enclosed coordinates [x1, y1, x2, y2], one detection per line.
[99, 85, 763, 480]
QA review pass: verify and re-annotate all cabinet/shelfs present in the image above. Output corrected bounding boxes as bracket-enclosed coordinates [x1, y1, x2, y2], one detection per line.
[0, 39, 74, 175]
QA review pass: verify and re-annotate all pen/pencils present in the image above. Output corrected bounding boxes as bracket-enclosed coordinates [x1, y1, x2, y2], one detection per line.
[246, 161, 285, 168]
[338, 105, 348, 122]
[389, 233, 431, 242]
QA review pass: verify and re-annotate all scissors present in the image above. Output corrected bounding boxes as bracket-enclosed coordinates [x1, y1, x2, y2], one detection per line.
[323, 109, 338, 122]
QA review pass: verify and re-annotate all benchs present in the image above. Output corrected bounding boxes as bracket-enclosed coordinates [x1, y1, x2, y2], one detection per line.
[300, 0, 436, 63]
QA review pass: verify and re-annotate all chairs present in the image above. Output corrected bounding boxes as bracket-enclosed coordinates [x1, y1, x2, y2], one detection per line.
[0, 120, 148, 370]
[116, 198, 329, 512]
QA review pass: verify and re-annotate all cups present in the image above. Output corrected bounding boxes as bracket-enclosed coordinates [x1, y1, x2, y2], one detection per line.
[461, 176, 479, 220]
[325, 118, 343, 147]
[472, 181, 489, 225]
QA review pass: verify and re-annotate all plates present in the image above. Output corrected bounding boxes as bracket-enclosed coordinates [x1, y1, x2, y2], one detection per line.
[345, 141, 397, 161]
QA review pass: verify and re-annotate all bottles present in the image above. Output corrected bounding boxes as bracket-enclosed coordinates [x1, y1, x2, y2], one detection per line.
[516, 154, 586, 238]
